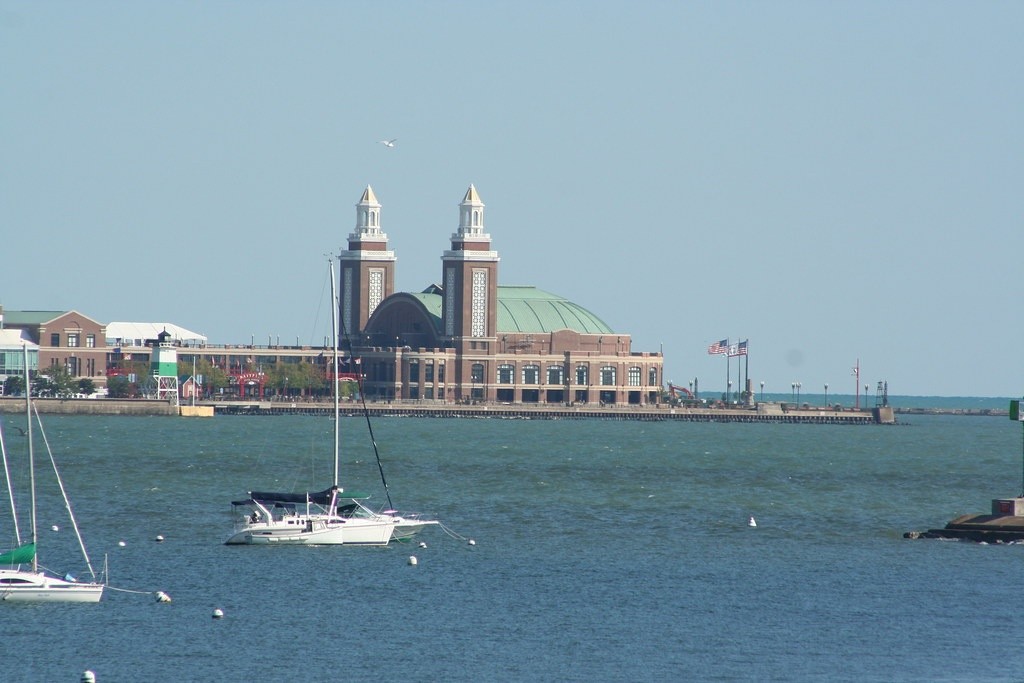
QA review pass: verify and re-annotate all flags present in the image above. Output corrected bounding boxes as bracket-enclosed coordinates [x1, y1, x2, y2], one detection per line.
[708, 339, 750, 357]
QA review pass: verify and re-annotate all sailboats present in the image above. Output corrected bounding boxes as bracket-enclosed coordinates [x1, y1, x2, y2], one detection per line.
[222, 260, 438, 546]
[1, 341, 107, 603]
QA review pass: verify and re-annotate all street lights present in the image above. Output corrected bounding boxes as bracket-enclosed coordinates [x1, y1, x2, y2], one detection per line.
[689, 380, 694, 394]
[864, 383, 868, 410]
[760, 381, 764, 401]
[791, 383, 795, 403]
[796, 383, 801, 404]
[824, 383, 828, 406]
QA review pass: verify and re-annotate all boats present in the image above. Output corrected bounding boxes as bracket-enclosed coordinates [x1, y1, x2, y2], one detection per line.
[902, 480, 1024, 540]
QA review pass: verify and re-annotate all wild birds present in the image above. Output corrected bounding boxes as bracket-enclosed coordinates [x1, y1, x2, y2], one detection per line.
[375, 138, 398, 147]
[12, 426, 27, 436]
